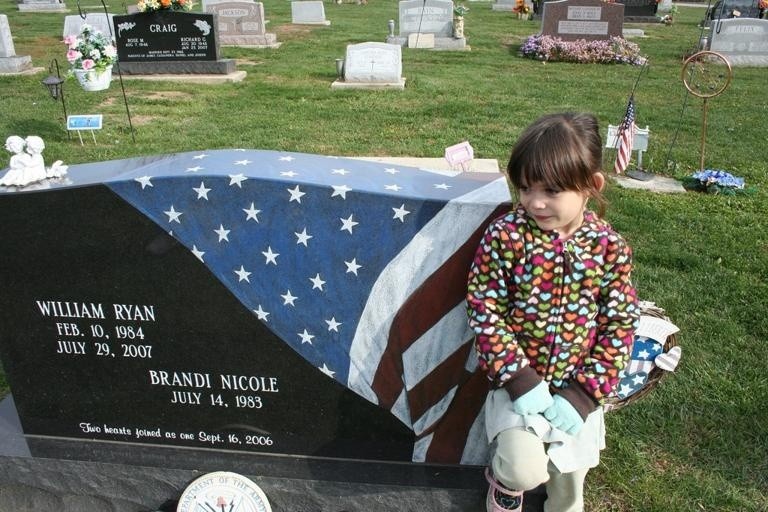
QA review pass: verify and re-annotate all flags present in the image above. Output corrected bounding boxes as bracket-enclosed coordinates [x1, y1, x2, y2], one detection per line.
[609, 95, 636, 177]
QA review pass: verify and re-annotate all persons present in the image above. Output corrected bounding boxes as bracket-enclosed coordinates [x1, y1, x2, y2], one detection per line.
[465, 113, 640, 512]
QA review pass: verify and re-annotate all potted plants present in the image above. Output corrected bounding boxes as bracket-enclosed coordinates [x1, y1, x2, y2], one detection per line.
[453, 5, 470, 39]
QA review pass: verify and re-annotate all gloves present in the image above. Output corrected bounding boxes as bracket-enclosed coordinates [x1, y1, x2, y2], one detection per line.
[543, 394, 583, 439]
[513, 378, 554, 419]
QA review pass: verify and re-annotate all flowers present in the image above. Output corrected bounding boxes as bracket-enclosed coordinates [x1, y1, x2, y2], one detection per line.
[60, 24, 119, 83]
[137, 0, 193, 13]
[512, 0, 534, 19]
[520, 32, 649, 68]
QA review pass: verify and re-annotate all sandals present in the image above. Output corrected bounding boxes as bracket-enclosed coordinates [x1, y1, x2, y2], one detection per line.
[484, 471, 524, 512]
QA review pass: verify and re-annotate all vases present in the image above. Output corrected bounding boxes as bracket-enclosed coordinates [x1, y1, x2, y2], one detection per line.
[74, 65, 114, 92]
[518, 13, 528, 22]
